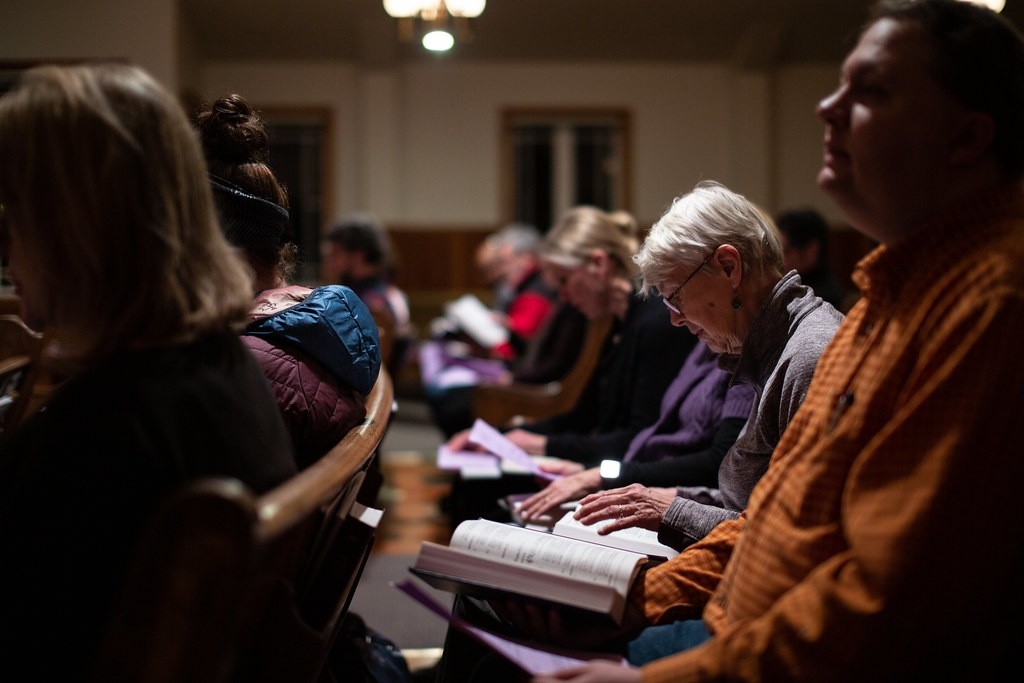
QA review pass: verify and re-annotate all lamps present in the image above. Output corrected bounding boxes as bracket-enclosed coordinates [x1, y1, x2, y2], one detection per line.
[382, 0, 488, 59]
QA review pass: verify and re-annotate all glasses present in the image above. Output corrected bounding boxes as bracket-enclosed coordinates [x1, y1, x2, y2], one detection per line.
[661, 250, 717, 313]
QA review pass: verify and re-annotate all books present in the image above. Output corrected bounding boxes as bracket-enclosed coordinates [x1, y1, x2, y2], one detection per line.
[409, 504, 678, 623]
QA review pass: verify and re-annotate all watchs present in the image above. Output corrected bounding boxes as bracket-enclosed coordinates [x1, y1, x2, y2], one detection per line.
[600, 459, 621, 487]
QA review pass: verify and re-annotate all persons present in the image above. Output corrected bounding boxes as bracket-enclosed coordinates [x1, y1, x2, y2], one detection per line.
[538, 0, 1024, 683]
[429, 182, 864, 553]
[0, 59, 409, 683]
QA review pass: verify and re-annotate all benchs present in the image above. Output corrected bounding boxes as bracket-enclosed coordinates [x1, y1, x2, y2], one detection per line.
[469, 309, 614, 431]
[141, 353, 400, 682]
